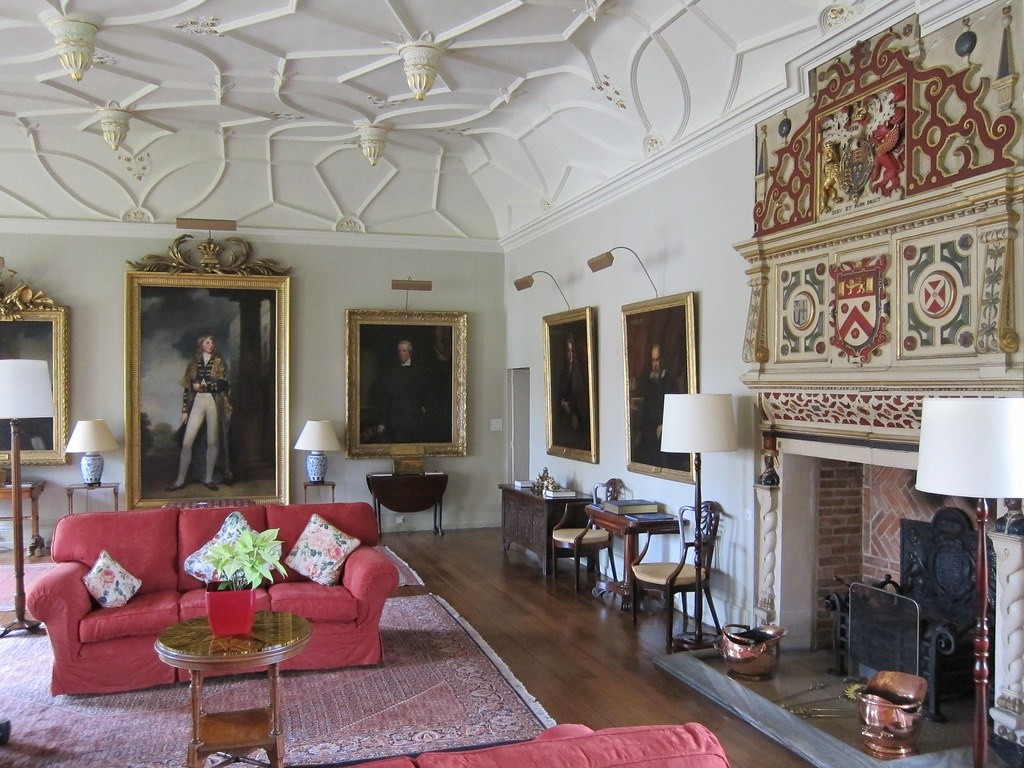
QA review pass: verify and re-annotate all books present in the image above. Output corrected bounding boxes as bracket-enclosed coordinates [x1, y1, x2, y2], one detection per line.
[546, 489, 576, 497]
[589, 499, 674, 522]
[515, 480, 534, 486]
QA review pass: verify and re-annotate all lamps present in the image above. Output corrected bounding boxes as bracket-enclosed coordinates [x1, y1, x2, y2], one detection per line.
[512, 268, 570, 315]
[293, 418, 342, 483]
[175, 216, 239, 271]
[659, 393, 737, 658]
[0, 357, 56, 637]
[390, 276, 431, 314]
[586, 245, 658, 297]
[64, 419, 118, 488]
[910, 396, 1022, 767]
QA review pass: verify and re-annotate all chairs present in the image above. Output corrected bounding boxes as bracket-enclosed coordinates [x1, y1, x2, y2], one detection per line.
[550, 477, 622, 592]
[628, 502, 727, 654]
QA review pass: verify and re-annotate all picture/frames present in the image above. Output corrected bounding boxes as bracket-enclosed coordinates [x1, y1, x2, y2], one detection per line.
[544, 305, 601, 463]
[345, 306, 469, 460]
[621, 293, 698, 484]
[124, 238, 291, 511]
[1, 258, 69, 467]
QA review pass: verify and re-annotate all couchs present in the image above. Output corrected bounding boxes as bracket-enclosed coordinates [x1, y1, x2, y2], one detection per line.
[353, 721, 729, 766]
[28, 501, 400, 700]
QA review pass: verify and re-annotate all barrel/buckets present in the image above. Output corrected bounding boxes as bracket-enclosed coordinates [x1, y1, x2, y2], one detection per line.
[722, 622, 787, 683]
[854, 672, 927, 759]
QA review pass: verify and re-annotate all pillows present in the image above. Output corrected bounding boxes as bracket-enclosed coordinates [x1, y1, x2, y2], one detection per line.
[84, 546, 140, 609]
[184, 511, 280, 588]
[286, 514, 362, 587]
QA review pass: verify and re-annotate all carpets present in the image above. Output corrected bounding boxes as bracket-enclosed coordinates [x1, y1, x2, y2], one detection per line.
[0, 592, 555, 768]
[1, 539, 425, 612]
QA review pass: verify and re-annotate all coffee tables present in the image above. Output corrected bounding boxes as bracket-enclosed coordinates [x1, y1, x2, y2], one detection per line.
[154, 616, 313, 764]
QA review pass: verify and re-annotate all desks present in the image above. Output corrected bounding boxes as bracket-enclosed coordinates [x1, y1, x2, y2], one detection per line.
[67, 481, 121, 514]
[0, 478, 47, 556]
[365, 473, 449, 538]
[582, 505, 677, 623]
[495, 482, 598, 580]
[304, 481, 337, 505]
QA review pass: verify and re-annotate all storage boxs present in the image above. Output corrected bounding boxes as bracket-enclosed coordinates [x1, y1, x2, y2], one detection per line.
[393, 459, 425, 474]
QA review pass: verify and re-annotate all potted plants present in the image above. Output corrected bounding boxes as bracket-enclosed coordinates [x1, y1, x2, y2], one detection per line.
[199, 525, 284, 637]
